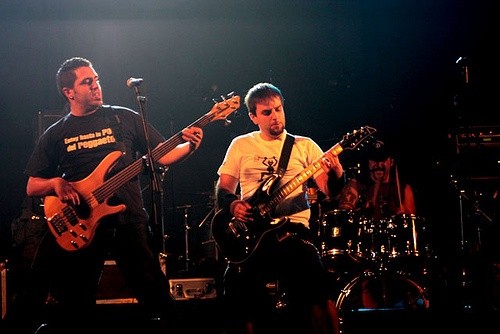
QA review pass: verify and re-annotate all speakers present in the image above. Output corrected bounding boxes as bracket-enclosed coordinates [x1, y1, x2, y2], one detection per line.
[94, 261, 138, 304]
[454, 131, 500, 264]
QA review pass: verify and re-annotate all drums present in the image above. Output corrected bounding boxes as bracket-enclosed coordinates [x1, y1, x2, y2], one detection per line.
[317, 208, 429, 271]
[336, 271, 430, 333]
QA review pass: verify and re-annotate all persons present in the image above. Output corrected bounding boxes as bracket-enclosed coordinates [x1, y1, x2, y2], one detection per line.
[215, 83, 345, 334]
[307, 178, 319, 204]
[338, 142, 416, 310]
[27, 57, 203, 334]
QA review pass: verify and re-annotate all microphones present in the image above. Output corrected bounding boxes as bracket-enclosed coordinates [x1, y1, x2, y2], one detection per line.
[126, 77, 145, 88]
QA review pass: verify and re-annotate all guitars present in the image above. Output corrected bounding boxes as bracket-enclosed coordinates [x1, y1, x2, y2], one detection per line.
[43, 89, 240, 251]
[210, 126, 380, 264]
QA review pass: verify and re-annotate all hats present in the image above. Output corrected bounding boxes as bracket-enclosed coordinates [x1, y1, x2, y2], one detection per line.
[367, 135, 393, 161]
